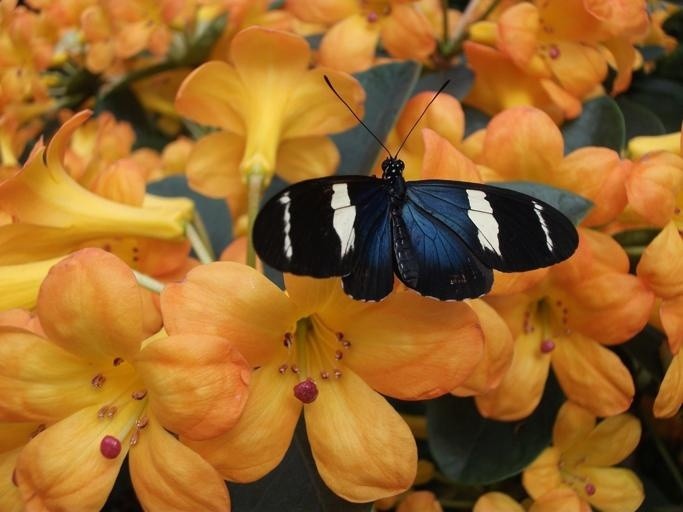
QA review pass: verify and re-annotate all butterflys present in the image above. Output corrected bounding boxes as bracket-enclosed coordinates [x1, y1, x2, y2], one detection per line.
[250, 73, 580, 306]
